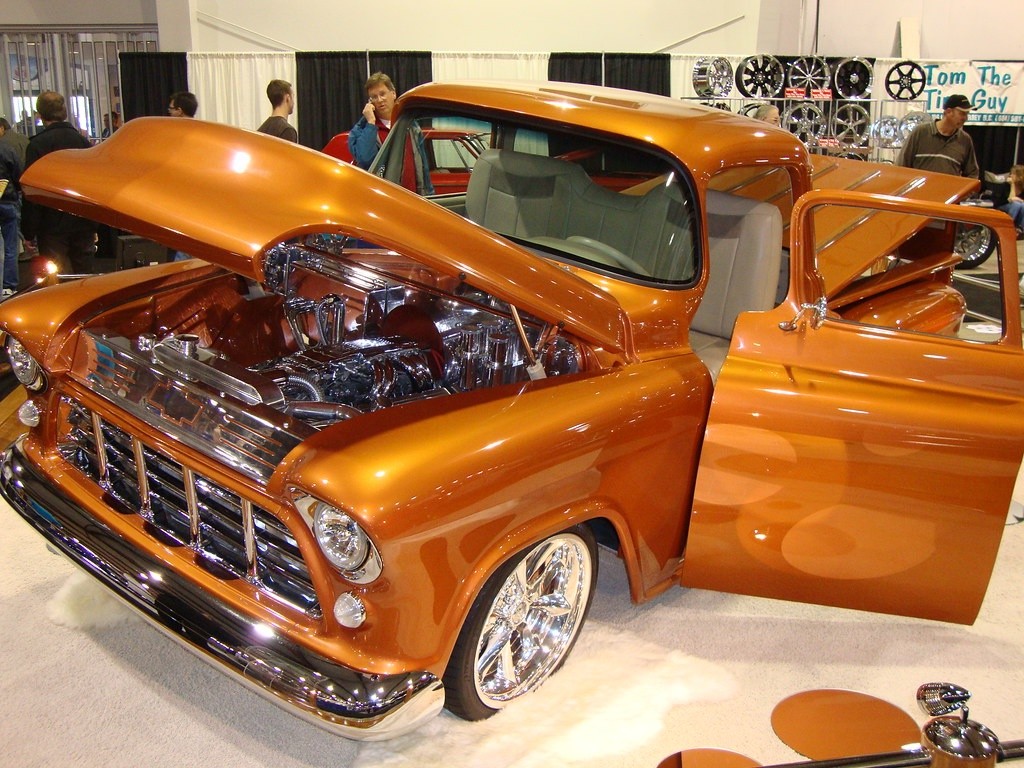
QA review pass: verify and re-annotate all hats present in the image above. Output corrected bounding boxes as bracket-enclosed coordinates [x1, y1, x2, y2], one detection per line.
[946, 94, 978, 112]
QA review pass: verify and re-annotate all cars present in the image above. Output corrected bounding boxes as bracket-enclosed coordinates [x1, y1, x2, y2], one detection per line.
[1, 78, 1024, 743]
[318, 130, 667, 224]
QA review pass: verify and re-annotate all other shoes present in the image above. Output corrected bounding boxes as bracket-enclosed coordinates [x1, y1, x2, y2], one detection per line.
[1015, 226, 1023, 239]
[1, 288, 18, 300]
[18, 240, 39, 260]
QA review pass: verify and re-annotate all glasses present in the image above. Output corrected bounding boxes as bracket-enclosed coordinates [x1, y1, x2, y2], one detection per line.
[168, 105, 178, 113]
[369, 90, 390, 103]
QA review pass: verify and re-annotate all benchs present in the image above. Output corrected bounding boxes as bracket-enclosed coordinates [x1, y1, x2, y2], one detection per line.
[466, 148, 782, 386]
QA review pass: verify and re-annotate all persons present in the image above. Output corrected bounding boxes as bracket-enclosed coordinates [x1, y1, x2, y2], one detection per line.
[102, 111, 119, 141]
[168, 91, 197, 262]
[0, 118, 31, 262]
[26, 92, 92, 169]
[257, 80, 297, 144]
[349, 72, 434, 193]
[897, 95, 979, 179]
[753, 105, 781, 127]
[996, 165, 1024, 228]
[0, 157, 19, 297]
